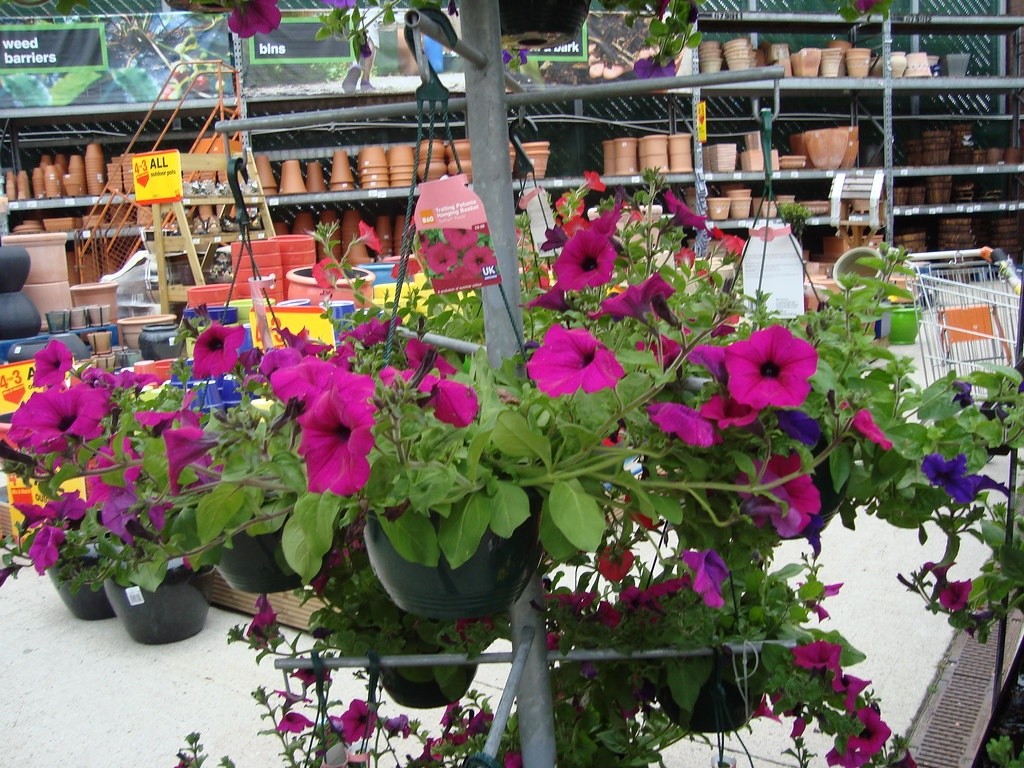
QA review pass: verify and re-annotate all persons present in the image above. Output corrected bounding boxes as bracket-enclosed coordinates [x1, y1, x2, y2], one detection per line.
[588, 42, 660, 80]
[342, 9, 379, 93]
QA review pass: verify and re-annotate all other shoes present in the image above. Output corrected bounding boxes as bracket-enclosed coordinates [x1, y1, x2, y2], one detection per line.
[361, 80, 375, 91]
[342, 66, 361, 93]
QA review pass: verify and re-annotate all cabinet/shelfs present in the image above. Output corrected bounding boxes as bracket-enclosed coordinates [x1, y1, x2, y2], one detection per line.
[683, 9, 1022, 229]
[6, 185, 256, 243]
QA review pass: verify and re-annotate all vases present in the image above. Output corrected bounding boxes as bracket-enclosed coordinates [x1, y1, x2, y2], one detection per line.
[16, 31, 1014, 368]
[23, 492, 770, 739]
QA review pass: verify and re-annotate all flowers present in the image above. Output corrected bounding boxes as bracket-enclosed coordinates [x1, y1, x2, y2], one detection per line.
[1, 162, 1023, 768]
